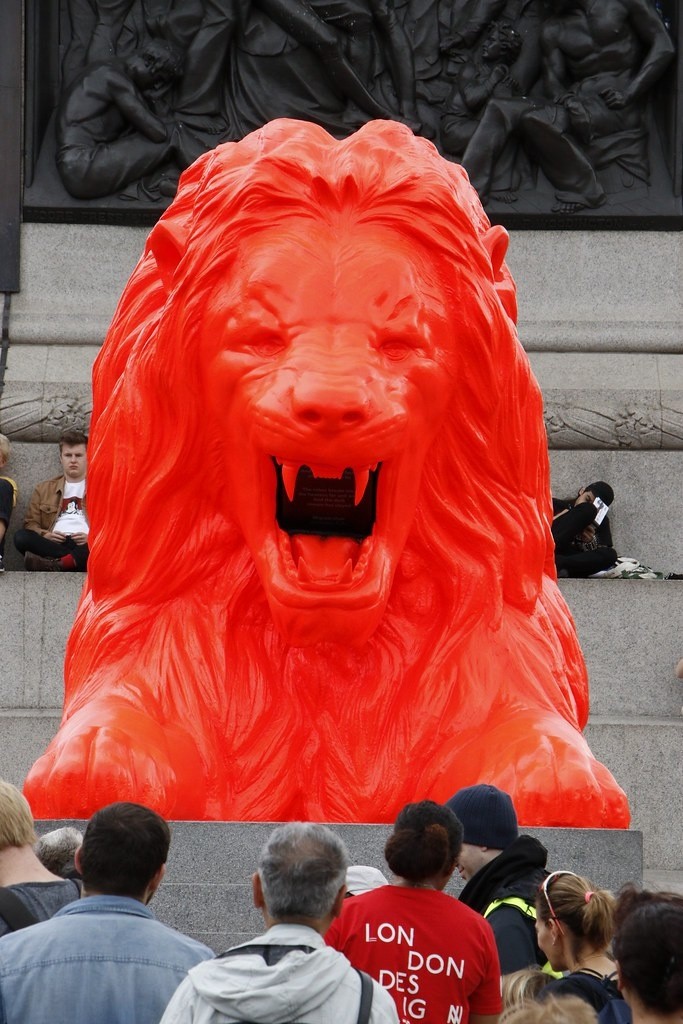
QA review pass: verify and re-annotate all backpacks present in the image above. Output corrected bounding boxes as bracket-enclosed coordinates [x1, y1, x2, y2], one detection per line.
[564, 975, 633, 1024]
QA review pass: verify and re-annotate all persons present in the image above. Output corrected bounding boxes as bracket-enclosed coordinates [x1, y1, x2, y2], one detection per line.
[443, 784, 551, 973]
[0, 432, 17, 574]
[13, 432, 89, 572]
[552, 481, 617, 578]
[0, 779, 84, 938]
[535, 870, 625, 1015]
[324, 800, 499, 1024]
[613, 882, 683, 1024]
[160, 821, 399, 1024]
[0, 800, 217, 1024]
[493, 966, 598, 1024]
[36, 826, 84, 881]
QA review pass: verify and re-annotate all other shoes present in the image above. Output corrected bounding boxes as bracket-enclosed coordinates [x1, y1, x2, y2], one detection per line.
[24, 552, 63, 571]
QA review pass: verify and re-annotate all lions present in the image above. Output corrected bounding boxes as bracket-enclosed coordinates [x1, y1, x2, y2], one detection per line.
[20, 118, 632, 830]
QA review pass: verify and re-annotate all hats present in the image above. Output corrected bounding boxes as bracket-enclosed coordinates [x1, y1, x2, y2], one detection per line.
[446, 784, 518, 850]
[343, 866, 388, 896]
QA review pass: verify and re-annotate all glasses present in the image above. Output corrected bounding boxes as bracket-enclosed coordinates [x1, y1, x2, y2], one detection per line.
[541, 869, 574, 935]
